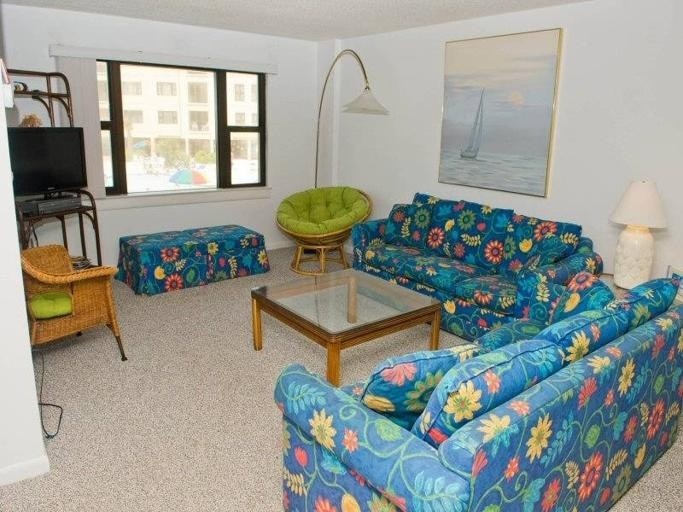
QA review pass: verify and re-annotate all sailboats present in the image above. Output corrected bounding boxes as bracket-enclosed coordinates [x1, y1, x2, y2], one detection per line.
[460, 87, 486, 161]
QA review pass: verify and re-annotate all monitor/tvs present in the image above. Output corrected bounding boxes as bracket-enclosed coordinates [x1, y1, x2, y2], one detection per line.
[7, 127, 88, 196]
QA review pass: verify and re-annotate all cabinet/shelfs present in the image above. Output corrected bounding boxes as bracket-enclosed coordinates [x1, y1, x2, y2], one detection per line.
[7, 70, 102, 270]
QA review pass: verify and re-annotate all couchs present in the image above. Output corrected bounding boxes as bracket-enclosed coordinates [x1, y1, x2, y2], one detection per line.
[351, 217, 603, 342]
[274, 305, 681, 512]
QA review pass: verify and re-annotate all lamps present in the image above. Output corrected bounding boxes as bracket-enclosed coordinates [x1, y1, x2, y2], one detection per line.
[314, 50, 388, 188]
[609, 180, 669, 291]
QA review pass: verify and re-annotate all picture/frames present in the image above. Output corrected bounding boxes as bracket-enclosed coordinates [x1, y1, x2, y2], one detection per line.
[437, 27, 562, 199]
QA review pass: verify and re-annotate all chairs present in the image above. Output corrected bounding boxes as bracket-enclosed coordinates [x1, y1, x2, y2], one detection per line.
[20, 244, 129, 361]
[274, 186, 372, 277]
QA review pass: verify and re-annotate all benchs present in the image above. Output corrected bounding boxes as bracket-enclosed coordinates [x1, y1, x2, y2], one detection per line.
[114, 224, 271, 295]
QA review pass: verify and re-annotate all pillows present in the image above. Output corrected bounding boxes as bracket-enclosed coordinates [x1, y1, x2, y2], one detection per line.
[533, 309, 619, 366]
[361, 341, 480, 415]
[383, 203, 431, 251]
[400, 193, 457, 254]
[447, 199, 514, 271]
[501, 214, 582, 283]
[549, 269, 616, 328]
[410, 337, 566, 447]
[605, 273, 680, 338]
